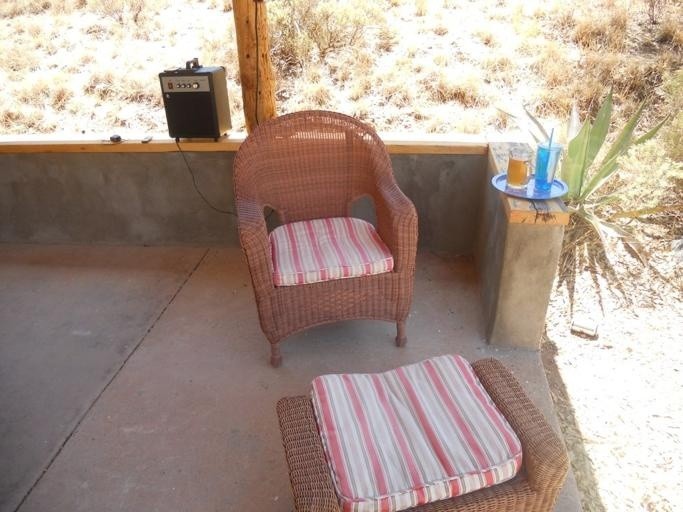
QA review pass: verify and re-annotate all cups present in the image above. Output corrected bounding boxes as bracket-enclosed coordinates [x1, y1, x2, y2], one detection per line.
[531, 142, 562, 191]
[506, 146, 531, 190]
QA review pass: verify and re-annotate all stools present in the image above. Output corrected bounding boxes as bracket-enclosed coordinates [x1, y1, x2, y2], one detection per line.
[276, 357, 569, 512]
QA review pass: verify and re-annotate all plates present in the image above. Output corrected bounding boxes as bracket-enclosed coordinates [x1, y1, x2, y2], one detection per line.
[491, 172, 567, 199]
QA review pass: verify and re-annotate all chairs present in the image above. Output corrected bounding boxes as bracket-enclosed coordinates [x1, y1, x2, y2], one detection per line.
[232, 109, 419, 369]
